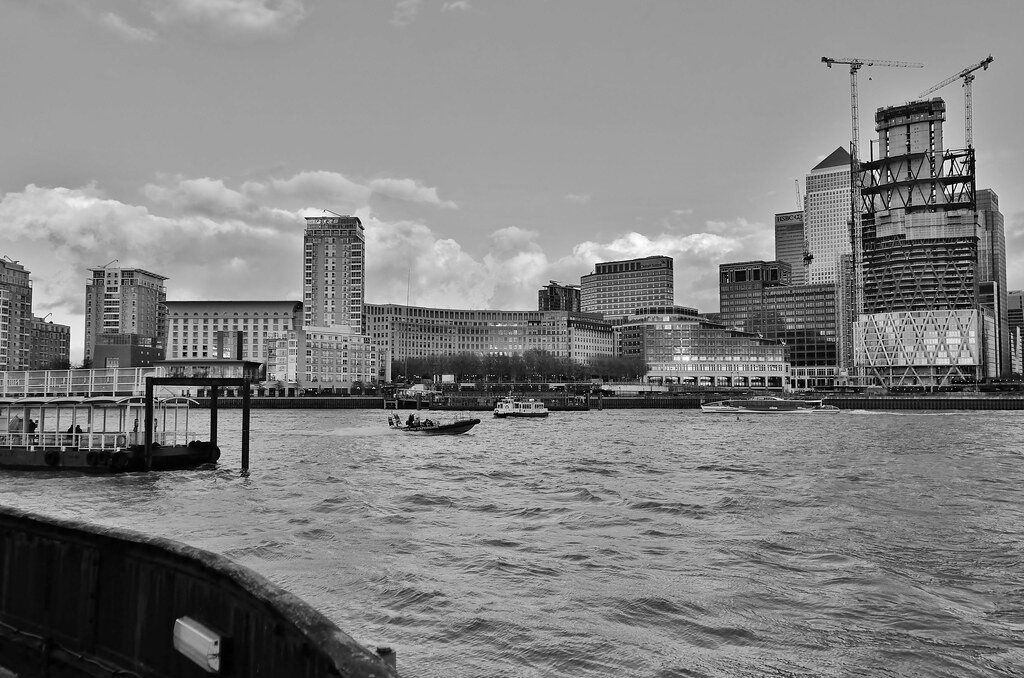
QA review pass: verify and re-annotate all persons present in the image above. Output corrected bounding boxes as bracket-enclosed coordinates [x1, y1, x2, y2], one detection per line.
[393, 412, 403, 426]
[29, 419, 36, 443]
[67, 425, 82, 445]
[407, 413, 434, 428]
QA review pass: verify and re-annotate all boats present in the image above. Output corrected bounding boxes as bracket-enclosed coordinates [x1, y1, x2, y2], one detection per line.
[701, 396, 841, 414]
[493, 397, 549, 418]
[388, 411, 481, 436]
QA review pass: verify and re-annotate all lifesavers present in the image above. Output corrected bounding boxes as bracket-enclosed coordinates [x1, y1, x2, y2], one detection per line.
[117, 435, 127, 447]
